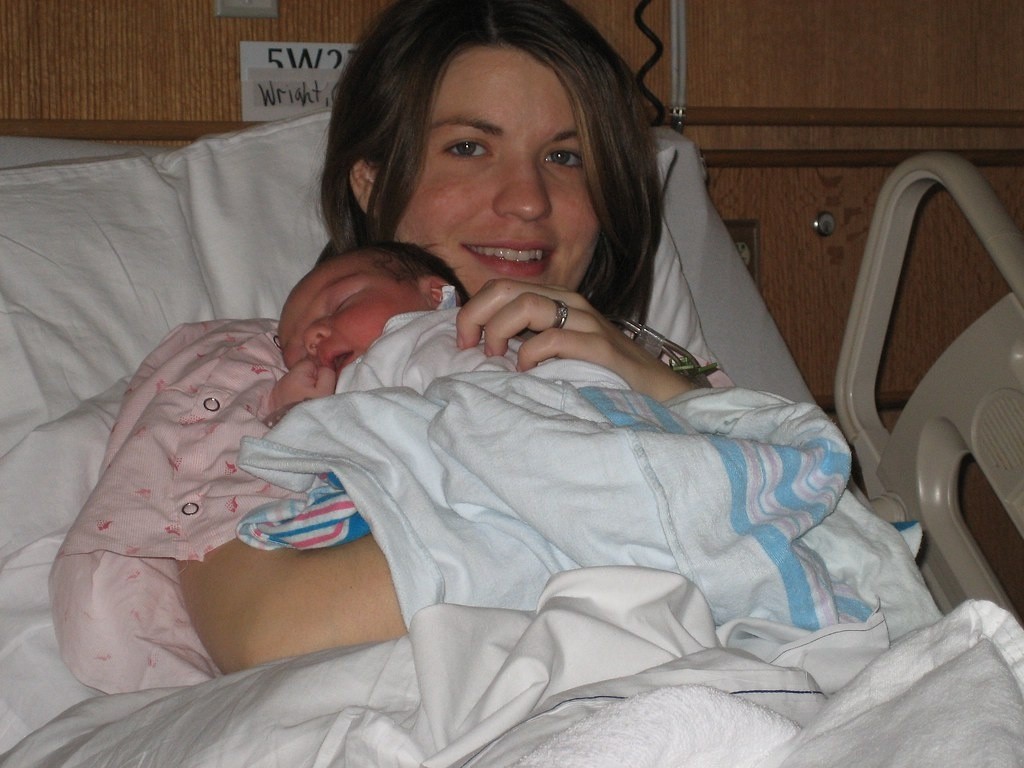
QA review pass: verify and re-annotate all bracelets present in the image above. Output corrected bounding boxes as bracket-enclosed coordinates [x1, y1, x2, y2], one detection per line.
[261, 397, 313, 430]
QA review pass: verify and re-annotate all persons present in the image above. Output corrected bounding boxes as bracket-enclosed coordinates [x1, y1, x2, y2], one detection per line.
[176, 0, 712, 675]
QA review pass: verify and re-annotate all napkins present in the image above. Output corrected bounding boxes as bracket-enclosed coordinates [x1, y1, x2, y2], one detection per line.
[1, 106, 732, 531]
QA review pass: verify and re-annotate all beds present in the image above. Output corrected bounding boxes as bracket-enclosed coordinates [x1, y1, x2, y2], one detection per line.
[0, 138, 1023, 768]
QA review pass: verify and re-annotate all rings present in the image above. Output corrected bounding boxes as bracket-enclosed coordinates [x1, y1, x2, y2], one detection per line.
[552, 300, 568, 329]
